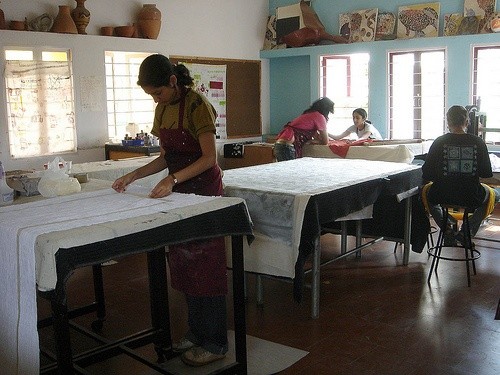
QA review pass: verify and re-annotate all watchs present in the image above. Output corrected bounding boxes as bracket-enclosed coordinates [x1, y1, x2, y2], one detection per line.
[171, 174, 179, 186]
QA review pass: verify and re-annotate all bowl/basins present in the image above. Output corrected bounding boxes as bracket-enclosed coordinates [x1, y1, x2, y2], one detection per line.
[114, 26, 135, 38]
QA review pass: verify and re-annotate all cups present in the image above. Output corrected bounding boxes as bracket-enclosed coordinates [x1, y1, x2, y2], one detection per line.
[10, 21, 25, 30]
[101, 27, 113, 36]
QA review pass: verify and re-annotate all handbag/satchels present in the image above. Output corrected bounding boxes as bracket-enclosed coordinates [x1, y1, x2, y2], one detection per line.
[38, 156, 81, 198]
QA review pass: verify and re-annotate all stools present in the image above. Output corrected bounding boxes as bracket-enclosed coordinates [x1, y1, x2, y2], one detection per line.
[427, 202, 481, 285]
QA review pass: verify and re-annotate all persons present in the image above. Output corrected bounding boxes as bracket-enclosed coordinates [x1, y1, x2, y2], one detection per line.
[273, 96, 336, 161]
[111, 54, 228, 366]
[421, 104, 493, 249]
[327, 108, 383, 145]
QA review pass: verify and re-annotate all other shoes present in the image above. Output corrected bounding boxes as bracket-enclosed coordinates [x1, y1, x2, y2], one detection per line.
[182, 345, 225, 367]
[172, 337, 196, 352]
[444, 229, 458, 247]
[455, 231, 475, 248]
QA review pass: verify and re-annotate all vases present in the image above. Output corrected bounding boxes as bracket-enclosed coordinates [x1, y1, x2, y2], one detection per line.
[72, 0, 91, 34]
[138, 4, 162, 39]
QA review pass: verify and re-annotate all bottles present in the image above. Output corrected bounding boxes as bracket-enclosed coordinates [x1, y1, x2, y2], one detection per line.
[70, 0, 90, 34]
[138, 4, 161, 39]
[124, 130, 160, 149]
[53, 5, 78, 34]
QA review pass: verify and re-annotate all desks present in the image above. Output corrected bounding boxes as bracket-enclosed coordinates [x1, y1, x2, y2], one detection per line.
[226, 145, 275, 169]
[301, 139, 435, 164]
[105, 144, 159, 159]
[222, 156, 423, 319]
[20, 153, 170, 187]
[0, 177, 256, 375]
